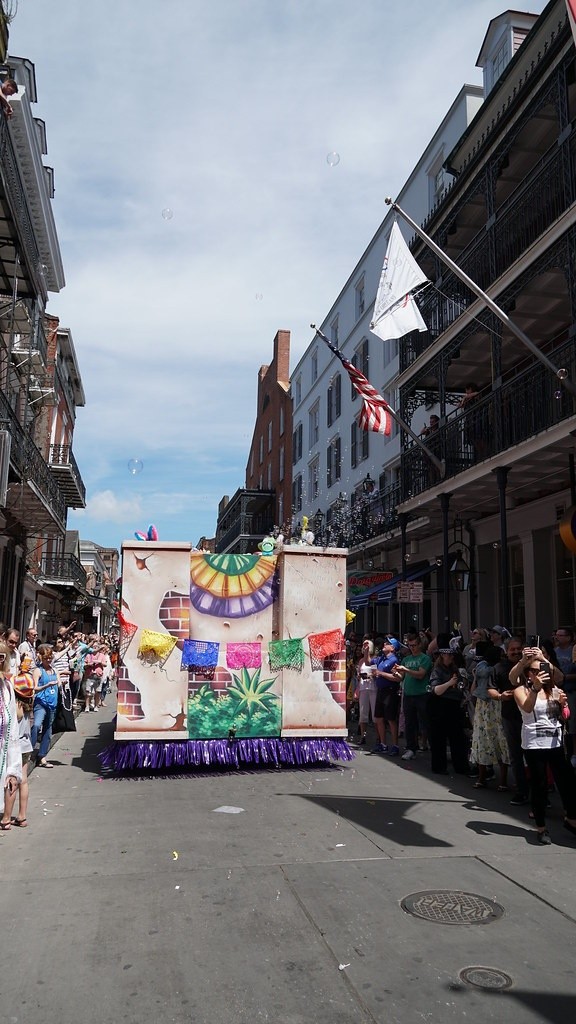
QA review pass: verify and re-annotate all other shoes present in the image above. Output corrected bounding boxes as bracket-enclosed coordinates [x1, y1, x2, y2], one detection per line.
[73, 701, 106, 712]
[345, 731, 576, 844]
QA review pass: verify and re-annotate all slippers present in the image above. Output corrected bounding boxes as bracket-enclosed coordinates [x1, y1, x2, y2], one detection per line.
[38, 762, 54, 768]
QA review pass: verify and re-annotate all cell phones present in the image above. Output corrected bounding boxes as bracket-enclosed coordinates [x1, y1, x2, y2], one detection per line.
[530, 635, 539, 648]
[540, 662, 550, 683]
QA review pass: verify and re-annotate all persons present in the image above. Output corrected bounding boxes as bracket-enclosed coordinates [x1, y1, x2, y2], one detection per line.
[0, 618, 119, 830]
[366, 484, 383, 520]
[419, 414, 448, 483]
[346, 621, 576, 846]
[457, 382, 495, 461]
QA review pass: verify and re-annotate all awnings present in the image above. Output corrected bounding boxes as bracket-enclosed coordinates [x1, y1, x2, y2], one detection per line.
[377, 565, 441, 602]
[350, 563, 427, 608]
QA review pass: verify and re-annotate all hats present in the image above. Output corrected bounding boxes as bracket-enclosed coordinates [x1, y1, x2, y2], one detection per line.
[486, 625, 507, 637]
[10, 673, 35, 698]
[425, 631, 436, 643]
[387, 637, 400, 652]
[437, 633, 461, 653]
[476, 627, 485, 640]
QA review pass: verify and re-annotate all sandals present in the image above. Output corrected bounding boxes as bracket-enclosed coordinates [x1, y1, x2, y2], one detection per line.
[11, 819, 27, 827]
[0, 821, 12, 830]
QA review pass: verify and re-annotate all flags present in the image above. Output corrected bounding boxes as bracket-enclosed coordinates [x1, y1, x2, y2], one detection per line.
[370, 221, 433, 342]
[316, 331, 396, 437]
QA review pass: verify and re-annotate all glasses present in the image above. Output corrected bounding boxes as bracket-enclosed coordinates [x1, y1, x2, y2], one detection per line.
[41, 654, 53, 661]
[58, 640, 64, 644]
[407, 644, 418, 648]
[527, 668, 541, 675]
[553, 633, 568, 639]
[0, 653, 6, 662]
[7, 639, 19, 645]
[471, 631, 479, 635]
[385, 641, 392, 645]
[29, 634, 38, 637]
